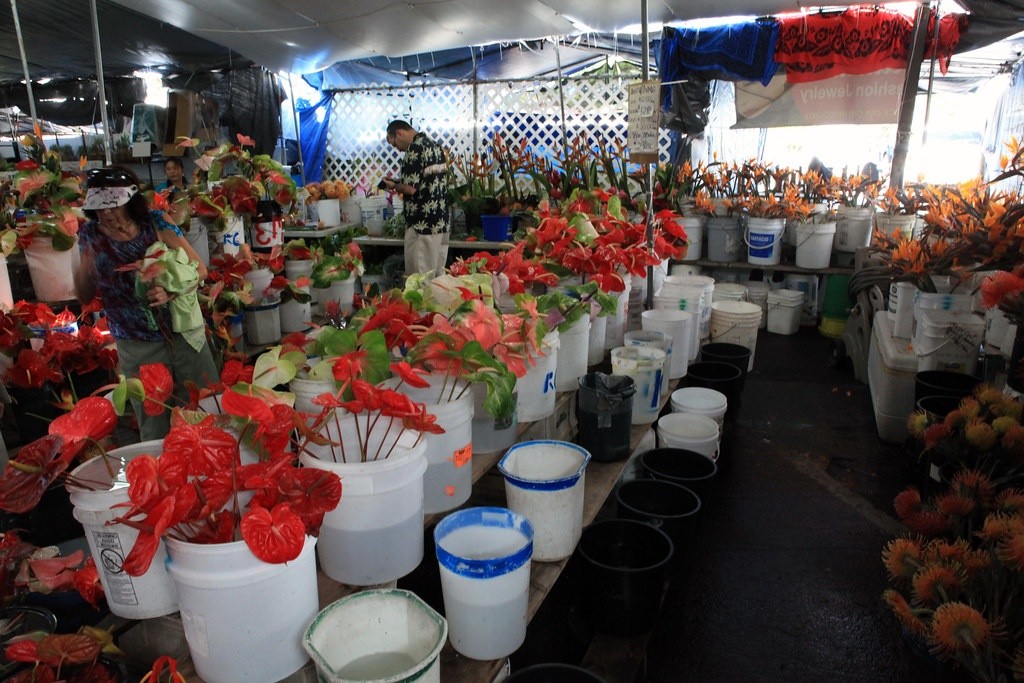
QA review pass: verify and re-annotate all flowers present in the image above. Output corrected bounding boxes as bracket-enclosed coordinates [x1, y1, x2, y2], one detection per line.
[0, 118, 1024, 683]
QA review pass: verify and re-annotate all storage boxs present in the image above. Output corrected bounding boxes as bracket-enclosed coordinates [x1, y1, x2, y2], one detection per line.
[868, 310, 977, 444]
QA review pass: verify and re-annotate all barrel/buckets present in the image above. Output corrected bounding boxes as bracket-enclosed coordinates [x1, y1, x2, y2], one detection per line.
[301, 587, 449, 683]
[498, 663, 610, 683]
[639, 447, 718, 501]
[577, 264, 988, 463]
[577, 518, 675, 639]
[433, 506, 536, 661]
[497, 439, 592, 562]
[615, 478, 703, 557]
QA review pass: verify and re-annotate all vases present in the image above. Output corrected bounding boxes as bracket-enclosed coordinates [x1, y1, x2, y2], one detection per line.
[244, 297, 281, 343]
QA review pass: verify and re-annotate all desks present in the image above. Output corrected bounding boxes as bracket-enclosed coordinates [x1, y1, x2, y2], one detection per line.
[352, 235, 517, 250]
[667, 259, 855, 277]
[284, 222, 361, 238]
[116, 358, 691, 683]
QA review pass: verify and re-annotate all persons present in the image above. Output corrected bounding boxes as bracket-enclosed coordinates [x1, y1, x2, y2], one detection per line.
[72, 164, 220, 442]
[154, 156, 190, 203]
[381, 120, 452, 288]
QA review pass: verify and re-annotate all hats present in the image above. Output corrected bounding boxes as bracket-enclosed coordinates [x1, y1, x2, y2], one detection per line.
[80, 184, 139, 214]
